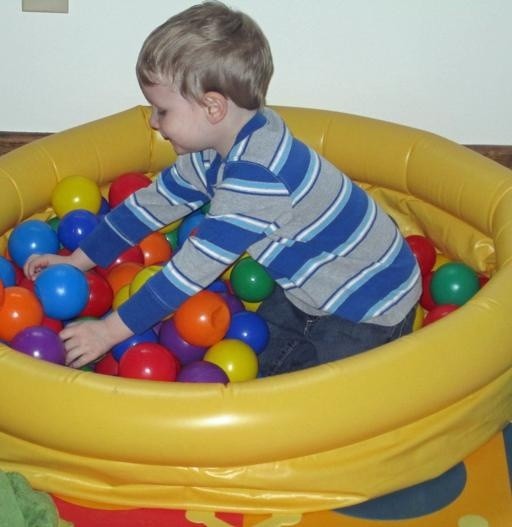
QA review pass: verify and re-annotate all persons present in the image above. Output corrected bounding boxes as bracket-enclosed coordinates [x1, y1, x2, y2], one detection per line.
[24, 1, 425, 379]
